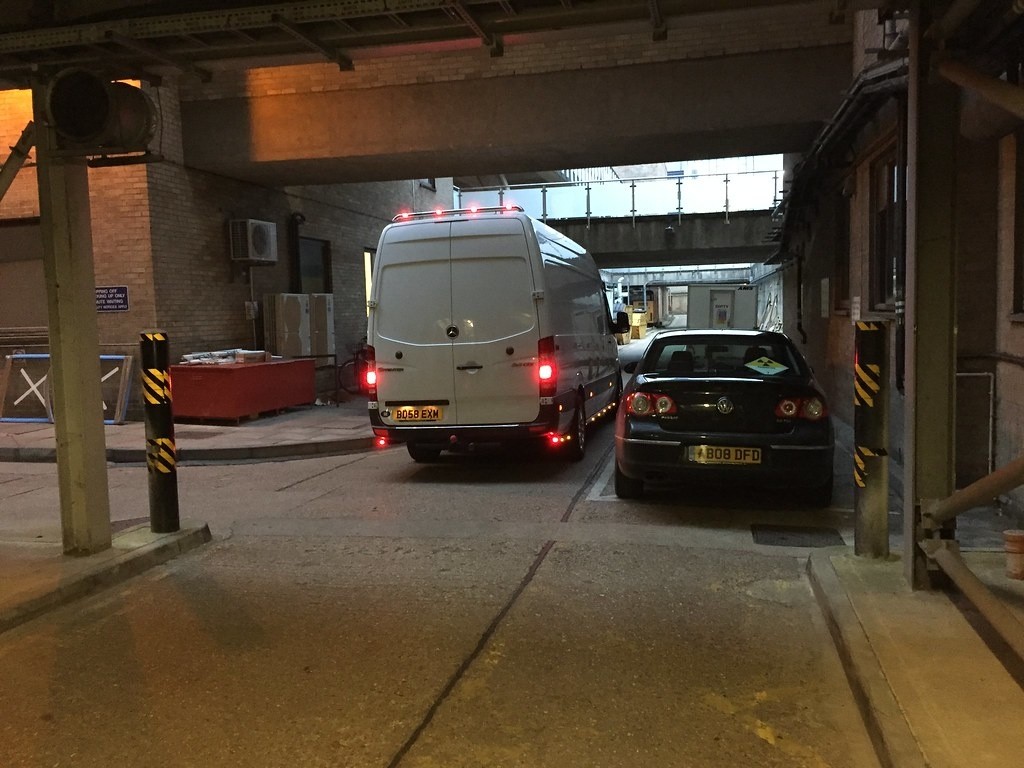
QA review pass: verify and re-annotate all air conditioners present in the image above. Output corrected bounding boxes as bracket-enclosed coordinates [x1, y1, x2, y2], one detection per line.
[231, 218, 278, 263]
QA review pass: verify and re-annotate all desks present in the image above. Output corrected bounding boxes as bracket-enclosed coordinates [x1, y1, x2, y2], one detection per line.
[168, 359, 317, 425]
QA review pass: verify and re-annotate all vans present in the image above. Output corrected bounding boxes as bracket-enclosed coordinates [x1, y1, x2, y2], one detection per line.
[366, 212, 620, 462]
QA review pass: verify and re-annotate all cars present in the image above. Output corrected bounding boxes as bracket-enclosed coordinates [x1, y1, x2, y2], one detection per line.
[612, 333, 834, 501]
